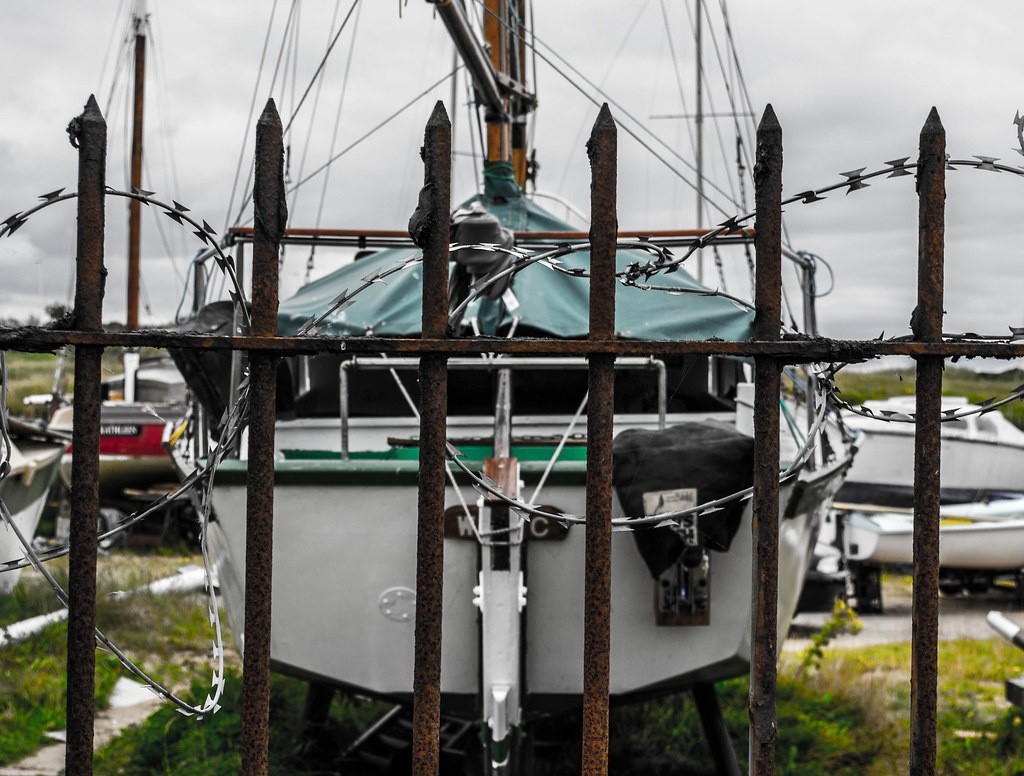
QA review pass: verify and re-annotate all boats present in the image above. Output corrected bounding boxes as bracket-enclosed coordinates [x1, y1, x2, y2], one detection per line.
[1, 1, 1024, 720]
